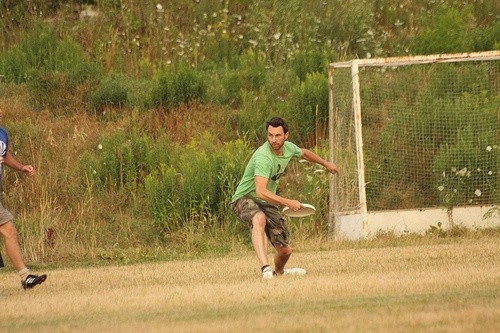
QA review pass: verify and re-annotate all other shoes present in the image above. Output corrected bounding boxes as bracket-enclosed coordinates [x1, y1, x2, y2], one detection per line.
[261, 266, 272, 279]
[271, 268, 306, 276]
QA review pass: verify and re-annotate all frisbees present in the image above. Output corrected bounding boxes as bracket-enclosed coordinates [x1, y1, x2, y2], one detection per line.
[282, 202, 316, 217]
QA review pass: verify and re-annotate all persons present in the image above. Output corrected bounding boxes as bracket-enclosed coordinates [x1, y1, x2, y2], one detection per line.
[0, 106, 47, 289]
[234, 117, 341, 279]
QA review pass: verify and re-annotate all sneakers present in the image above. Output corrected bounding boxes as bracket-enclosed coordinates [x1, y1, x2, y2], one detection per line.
[20, 273, 48, 290]
